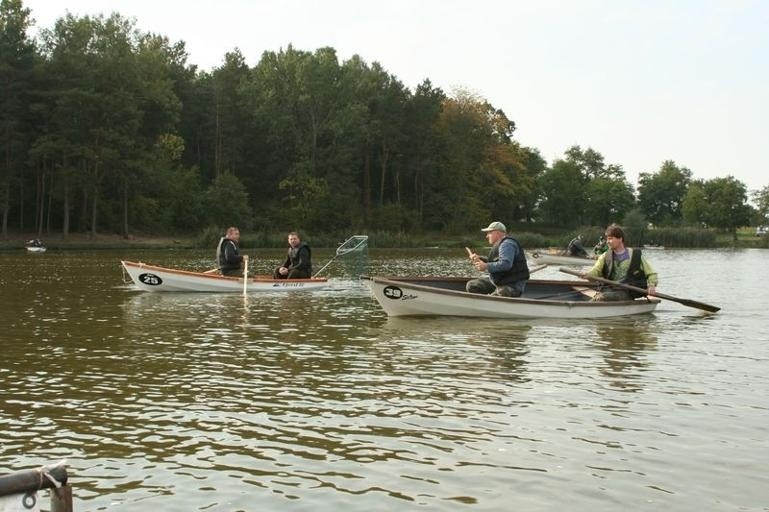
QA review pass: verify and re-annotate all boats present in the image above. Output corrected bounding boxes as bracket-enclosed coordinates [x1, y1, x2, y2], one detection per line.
[525, 247, 602, 268]
[358, 272, 664, 320]
[122, 259, 332, 294]
[24, 239, 49, 255]
[644, 243, 667, 250]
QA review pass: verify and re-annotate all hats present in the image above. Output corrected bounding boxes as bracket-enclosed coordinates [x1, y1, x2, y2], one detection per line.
[480, 221, 507, 234]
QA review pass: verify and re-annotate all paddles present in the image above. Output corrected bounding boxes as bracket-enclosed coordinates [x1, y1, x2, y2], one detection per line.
[464, 246, 479, 261]
[559, 267, 721, 313]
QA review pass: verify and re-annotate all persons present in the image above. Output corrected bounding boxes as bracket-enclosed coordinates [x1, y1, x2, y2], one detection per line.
[465, 222, 530, 297]
[568, 233, 589, 257]
[592, 236, 609, 260]
[272, 231, 312, 279]
[581, 224, 658, 301]
[216, 227, 254, 278]
